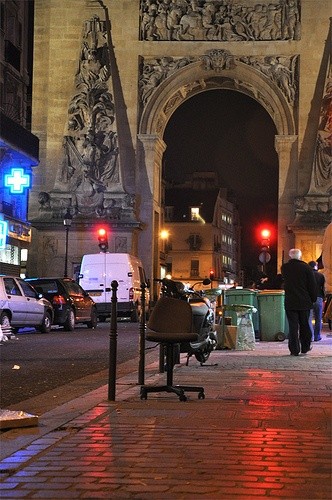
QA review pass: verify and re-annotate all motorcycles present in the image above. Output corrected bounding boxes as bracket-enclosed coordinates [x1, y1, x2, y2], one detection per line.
[153, 277, 219, 371]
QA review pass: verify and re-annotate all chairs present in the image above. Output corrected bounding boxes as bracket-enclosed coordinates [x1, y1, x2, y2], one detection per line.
[140, 298, 206, 402]
[35, 287, 43, 294]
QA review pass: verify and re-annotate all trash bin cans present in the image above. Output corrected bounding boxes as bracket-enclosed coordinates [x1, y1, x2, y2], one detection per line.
[224, 288, 289, 339]
[256, 289, 285, 342]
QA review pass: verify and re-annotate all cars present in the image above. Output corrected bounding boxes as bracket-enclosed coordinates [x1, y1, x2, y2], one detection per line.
[0, 275, 56, 334]
[23, 276, 98, 331]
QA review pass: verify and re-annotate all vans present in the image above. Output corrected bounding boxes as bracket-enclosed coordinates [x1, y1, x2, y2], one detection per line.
[78, 252, 151, 322]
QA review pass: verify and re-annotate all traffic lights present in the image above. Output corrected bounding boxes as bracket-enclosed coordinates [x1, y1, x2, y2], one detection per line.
[98, 228, 109, 252]
[210, 270, 214, 280]
[260, 229, 270, 253]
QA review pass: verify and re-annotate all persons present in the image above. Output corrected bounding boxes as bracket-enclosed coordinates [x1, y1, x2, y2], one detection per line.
[256, 57, 293, 103]
[142, 57, 182, 102]
[229, 282, 238, 288]
[307, 261, 326, 342]
[139, 0, 299, 41]
[280, 248, 317, 355]
[259, 273, 278, 289]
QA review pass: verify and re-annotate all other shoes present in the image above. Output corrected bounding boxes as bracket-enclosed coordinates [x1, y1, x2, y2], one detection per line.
[301, 345, 313, 353]
[290, 351, 298, 355]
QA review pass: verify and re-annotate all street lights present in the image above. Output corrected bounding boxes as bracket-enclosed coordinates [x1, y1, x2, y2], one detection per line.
[63, 208, 73, 277]
